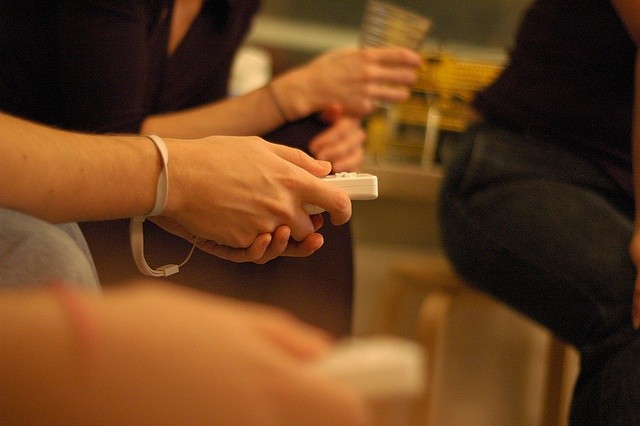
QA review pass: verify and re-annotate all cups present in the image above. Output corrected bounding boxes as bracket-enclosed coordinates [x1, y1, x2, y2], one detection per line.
[357, 1, 432, 47]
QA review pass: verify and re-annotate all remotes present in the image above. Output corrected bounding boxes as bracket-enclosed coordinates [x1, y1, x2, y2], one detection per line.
[298, 171, 379, 214]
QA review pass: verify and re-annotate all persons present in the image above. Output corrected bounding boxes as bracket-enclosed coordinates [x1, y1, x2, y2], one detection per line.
[1, 111, 354, 303]
[437, 2, 639, 426]
[0, 2, 431, 338]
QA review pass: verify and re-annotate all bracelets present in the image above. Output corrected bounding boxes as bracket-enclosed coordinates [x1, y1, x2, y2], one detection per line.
[267, 84, 288, 123]
